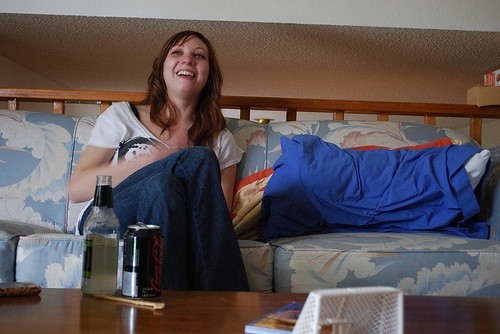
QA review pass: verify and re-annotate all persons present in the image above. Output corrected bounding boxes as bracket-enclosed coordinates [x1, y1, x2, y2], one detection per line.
[70, 30, 250, 293]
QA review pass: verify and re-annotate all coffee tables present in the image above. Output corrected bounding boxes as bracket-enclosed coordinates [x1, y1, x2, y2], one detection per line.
[0, 288, 500, 334]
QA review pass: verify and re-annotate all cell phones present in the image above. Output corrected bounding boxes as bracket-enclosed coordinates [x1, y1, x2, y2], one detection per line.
[0, 282, 42, 296]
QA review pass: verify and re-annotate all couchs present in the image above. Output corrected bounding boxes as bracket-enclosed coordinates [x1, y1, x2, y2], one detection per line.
[0, 109, 500, 298]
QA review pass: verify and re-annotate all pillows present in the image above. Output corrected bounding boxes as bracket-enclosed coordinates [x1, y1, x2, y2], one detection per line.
[257, 132, 494, 245]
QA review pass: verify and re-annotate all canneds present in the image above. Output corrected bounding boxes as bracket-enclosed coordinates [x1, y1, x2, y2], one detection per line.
[123, 222, 164, 298]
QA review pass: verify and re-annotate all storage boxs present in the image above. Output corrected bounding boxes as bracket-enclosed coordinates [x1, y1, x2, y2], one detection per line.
[483, 69, 500, 86]
[466, 84, 500, 108]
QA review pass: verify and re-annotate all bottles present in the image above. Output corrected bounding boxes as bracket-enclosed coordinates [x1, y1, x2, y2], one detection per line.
[81, 174, 119, 294]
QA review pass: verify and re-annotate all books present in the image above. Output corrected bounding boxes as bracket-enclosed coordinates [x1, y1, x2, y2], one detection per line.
[245, 301, 306, 334]
